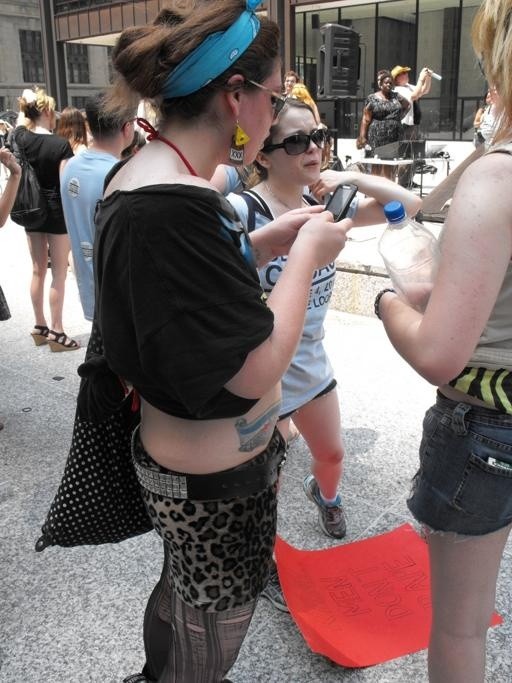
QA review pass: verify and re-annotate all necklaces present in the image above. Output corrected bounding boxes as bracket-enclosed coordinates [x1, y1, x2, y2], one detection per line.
[264, 182, 293, 210]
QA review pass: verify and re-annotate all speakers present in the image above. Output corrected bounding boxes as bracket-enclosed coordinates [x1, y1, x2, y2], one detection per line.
[316, 24, 360, 102]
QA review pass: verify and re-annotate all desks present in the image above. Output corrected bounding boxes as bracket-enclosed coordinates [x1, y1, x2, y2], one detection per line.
[349, 154, 451, 200]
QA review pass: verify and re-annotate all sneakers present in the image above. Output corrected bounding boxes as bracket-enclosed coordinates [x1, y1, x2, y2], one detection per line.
[303, 474, 346, 539]
[258, 558, 289, 612]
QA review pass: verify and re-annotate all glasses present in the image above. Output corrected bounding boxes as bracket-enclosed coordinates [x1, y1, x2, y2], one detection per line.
[261, 129, 325, 155]
[247, 78, 288, 120]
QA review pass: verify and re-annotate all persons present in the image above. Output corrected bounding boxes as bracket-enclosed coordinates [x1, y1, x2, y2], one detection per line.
[35, 0, 355, 683]
[375, 0, 512, 683]
[282, 65, 498, 188]
[225, 95, 422, 614]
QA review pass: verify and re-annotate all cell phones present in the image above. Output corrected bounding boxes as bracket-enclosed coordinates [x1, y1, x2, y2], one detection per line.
[323, 182, 359, 223]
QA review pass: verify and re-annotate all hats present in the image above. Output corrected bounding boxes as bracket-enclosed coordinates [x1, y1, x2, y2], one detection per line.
[391, 65, 412, 81]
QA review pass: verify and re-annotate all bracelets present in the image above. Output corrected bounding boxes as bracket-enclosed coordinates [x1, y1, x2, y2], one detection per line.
[374, 287, 397, 321]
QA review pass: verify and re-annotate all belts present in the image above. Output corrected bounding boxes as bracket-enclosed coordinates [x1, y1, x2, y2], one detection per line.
[131, 423, 289, 500]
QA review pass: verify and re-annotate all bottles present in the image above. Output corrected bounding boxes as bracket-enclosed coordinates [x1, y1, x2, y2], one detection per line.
[380, 199, 442, 312]
[363, 143, 372, 150]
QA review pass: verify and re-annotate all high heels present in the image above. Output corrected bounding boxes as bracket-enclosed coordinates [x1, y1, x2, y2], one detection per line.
[31, 326, 49, 346]
[46, 330, 79, 352]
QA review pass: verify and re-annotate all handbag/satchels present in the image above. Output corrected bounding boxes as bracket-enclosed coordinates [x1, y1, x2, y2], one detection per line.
[11, 161, 48, 226]
[36, 319, 154, 552]
